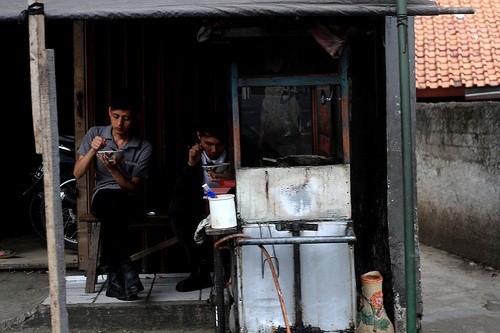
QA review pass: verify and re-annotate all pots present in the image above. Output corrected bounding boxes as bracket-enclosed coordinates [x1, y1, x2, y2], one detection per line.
[262, 155, 336, 167]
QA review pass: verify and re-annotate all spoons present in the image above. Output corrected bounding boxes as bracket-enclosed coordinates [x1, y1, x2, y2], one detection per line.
[104, 141, 117, 151]
[202, 150, 214, 164]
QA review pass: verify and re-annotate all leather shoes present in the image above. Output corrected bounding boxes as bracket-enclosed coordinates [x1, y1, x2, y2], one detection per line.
[106, 278, 136, 300]
[121, 264, 142, 296]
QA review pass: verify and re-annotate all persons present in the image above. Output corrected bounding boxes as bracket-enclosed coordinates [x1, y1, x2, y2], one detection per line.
[172, 120, 258, 292]
[73, 92, 153, 302]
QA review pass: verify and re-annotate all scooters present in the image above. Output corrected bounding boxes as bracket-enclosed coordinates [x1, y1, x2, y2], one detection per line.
[14, 130, 78, 251]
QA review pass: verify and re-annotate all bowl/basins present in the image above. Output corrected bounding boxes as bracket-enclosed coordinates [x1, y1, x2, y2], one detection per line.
[97, 150, 122, 162]
[202, 162, 230, 174]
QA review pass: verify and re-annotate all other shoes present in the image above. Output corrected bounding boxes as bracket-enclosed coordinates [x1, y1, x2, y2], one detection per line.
[176, 273, 212, 293]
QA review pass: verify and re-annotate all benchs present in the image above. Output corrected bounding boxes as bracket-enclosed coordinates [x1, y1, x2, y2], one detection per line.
[79, 213, 179, 292]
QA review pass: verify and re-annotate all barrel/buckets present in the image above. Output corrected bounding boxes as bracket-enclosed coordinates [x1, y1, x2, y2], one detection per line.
[208, 194, 238, 229]
